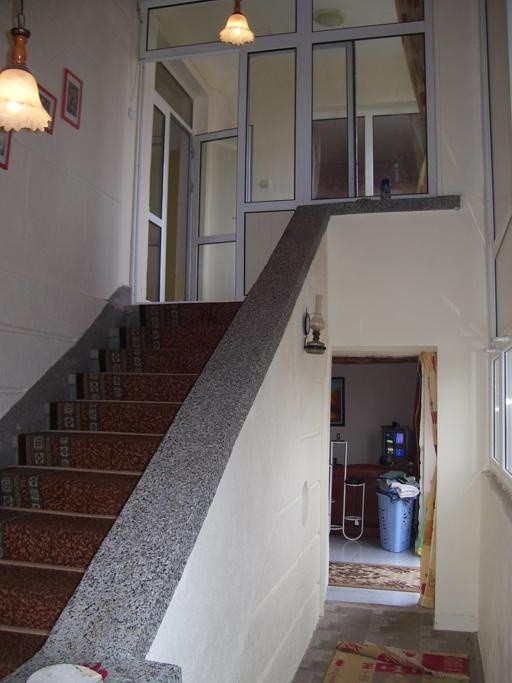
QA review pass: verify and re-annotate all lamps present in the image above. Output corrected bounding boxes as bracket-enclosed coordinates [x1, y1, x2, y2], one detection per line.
[303, 295, 329, 355]
[0, 0, 52, 133]
[219, 0, 256, 46]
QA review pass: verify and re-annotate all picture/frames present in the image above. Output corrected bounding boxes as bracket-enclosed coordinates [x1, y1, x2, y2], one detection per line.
[0, 67, 84, 171]
[330, 377, 345, 426]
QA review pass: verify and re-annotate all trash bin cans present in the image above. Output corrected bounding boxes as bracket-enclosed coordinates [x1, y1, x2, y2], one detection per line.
[376, 492, 414, 553]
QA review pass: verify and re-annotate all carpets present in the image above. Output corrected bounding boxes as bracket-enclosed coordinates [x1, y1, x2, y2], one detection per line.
[328, 562, 421, 593]
[322, 641, 470, 682]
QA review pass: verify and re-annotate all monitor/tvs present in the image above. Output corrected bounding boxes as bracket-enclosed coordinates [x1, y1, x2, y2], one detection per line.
[381, 428, 415, 461]
